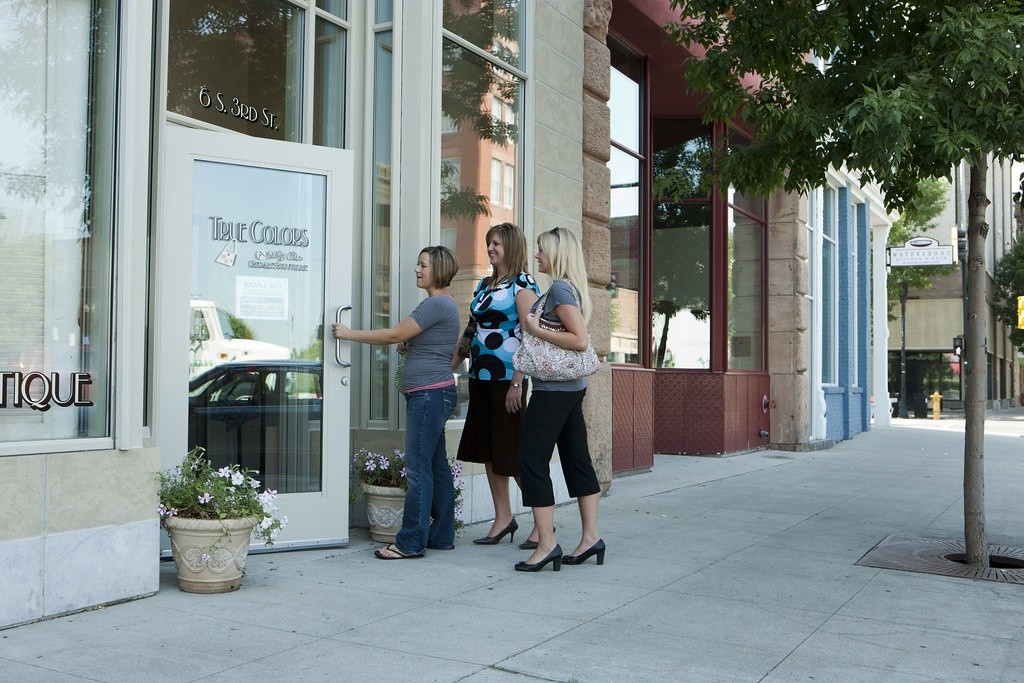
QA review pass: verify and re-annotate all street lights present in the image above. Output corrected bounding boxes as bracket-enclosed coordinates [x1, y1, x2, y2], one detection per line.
[896, 274, 908, 417]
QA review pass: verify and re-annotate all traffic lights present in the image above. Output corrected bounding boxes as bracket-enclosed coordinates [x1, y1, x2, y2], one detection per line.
[609, 272, 619, 298]
[953, 337, 963, 356]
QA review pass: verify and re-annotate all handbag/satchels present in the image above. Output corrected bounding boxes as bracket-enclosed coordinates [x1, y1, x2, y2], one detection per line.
[458, 276, 491, 358]
[512, 280, 601, 381]
[394, 342, 408, 393]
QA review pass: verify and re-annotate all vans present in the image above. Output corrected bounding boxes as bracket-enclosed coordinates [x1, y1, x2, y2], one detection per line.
[188, 299, 295, 394]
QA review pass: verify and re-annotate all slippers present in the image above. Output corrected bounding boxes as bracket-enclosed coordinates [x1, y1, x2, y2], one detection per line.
[375, 543, 424, 559]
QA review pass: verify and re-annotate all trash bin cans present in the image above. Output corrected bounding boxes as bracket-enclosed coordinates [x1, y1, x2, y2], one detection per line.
[913, 392, 928, 417]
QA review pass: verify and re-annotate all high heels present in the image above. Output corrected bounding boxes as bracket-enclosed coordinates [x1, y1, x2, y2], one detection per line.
[515, 543, 563, 572]
[519, 525, 556, 549]
[472, 516, 518, 545]
[562, 539, 605, 565]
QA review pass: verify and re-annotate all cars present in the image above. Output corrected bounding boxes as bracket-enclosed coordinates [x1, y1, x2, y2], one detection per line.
[188, 361, 325, 475]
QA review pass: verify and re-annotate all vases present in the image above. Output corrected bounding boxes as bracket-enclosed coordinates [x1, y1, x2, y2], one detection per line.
[164, 514, 253, 594]
[361, 483, 408, 543]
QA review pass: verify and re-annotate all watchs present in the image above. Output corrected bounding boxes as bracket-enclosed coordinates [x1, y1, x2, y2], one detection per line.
[510, 382, 522, 388]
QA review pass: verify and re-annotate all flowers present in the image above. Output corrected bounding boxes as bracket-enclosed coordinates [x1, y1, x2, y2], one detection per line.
[349, 448, 468, 534]
[156, 438, 290, 547]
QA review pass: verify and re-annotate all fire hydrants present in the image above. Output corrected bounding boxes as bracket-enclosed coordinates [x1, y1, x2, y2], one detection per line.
[930, 391, 943, 420]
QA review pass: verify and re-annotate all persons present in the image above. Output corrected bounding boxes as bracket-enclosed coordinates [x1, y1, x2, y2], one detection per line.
[514, 227, 606, 572]
[330, 246, 458, 560]
[449, 222, 557, 549]
